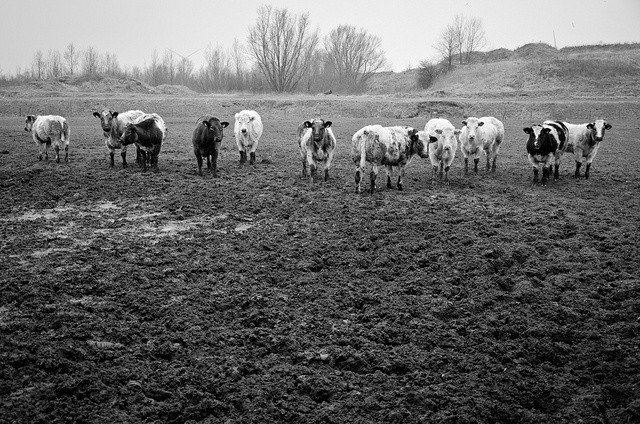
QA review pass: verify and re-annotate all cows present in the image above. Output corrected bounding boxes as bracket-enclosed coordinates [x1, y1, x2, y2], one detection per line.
[93, 110, 146, 168]
[459, 117, 504, 178]
[543, 120, 612, 182]
[297, 118, 336, 183]
[192, 114, 229, 178]
[424, 118, 462, 186]
[352, 125, 438, 195]
[119, 113, 168, 173]
[24, 115, 70, 163]
[522, 120, 569, 190]
[234, 110, 263, 165]
